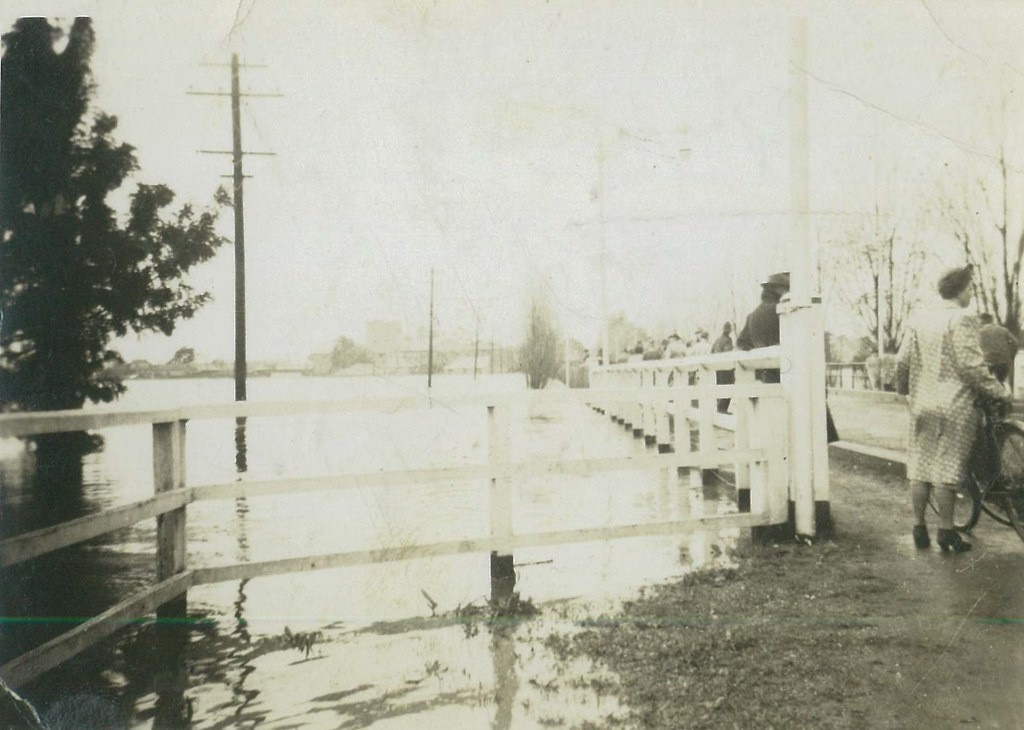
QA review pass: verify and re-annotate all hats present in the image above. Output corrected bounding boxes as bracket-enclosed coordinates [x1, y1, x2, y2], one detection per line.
[668, 333, 680, 339]
[938, 262, 973, 298]
[760, 272, 790, 289]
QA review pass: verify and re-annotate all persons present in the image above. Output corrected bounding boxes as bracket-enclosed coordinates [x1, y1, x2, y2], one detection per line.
[868, 339, 897, 391]
[980, 313, 1019, 385]
[582, 273, 838, 524]
[896, 264, 1013, 553]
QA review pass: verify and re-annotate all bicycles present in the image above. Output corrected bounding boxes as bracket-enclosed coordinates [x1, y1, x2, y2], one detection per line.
[922, 392, 1024, 540]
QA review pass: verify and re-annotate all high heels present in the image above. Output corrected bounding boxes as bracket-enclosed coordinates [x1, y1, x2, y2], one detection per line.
[937, 529, 972, 551]
[912, 525, 930, 547]
